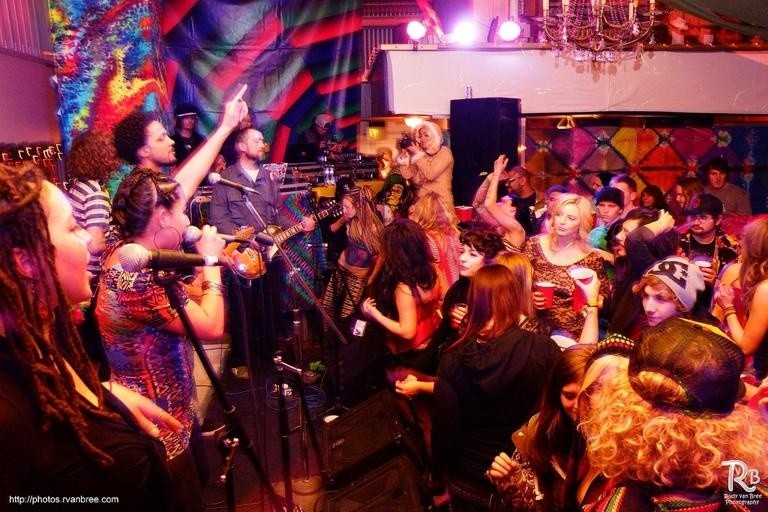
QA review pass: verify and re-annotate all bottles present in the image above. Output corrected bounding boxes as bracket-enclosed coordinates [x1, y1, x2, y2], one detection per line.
[314, 147, 378, 186]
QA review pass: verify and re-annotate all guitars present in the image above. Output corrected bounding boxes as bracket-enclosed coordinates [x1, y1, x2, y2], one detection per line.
[222, 199, 344, 280]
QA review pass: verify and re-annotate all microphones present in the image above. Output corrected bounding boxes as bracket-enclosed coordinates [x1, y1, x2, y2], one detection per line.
[119, 244, 229, 272]
[208, 172, 262, 195]
[183, 226, 251, 243]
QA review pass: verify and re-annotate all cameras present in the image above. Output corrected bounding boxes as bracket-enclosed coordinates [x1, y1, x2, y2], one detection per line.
[400, 133, 416, 148]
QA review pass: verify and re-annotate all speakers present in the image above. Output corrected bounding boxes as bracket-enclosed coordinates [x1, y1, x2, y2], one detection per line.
[328, 455, 435, 512]
[328, 390, 429, 483]
[450, 98, 522, 206]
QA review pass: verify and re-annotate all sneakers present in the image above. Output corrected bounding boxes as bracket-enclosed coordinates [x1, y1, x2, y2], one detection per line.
[229, 365, 252, 381]
[197, 419, 228, 440]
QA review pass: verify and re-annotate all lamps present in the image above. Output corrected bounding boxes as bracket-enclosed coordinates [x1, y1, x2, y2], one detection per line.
[531, 1, 657, 72]
[397, 19, 526, 54]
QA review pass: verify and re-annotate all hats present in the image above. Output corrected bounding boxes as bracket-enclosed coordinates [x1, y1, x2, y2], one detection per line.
[646, 255, 708, 313]
[626, 314, 749, 416]
[174, 103, 199, 119]
[594, 185, 625, 209]
[313, 113, 333, 130]
[677, 191, 724, 215]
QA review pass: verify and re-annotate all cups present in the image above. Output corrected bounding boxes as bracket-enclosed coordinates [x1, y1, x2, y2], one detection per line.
[536, 282, 557, 310]
[570, 267, 596, 288]
[694, 261, 712, 286]
[449, 303, 469, 330]
